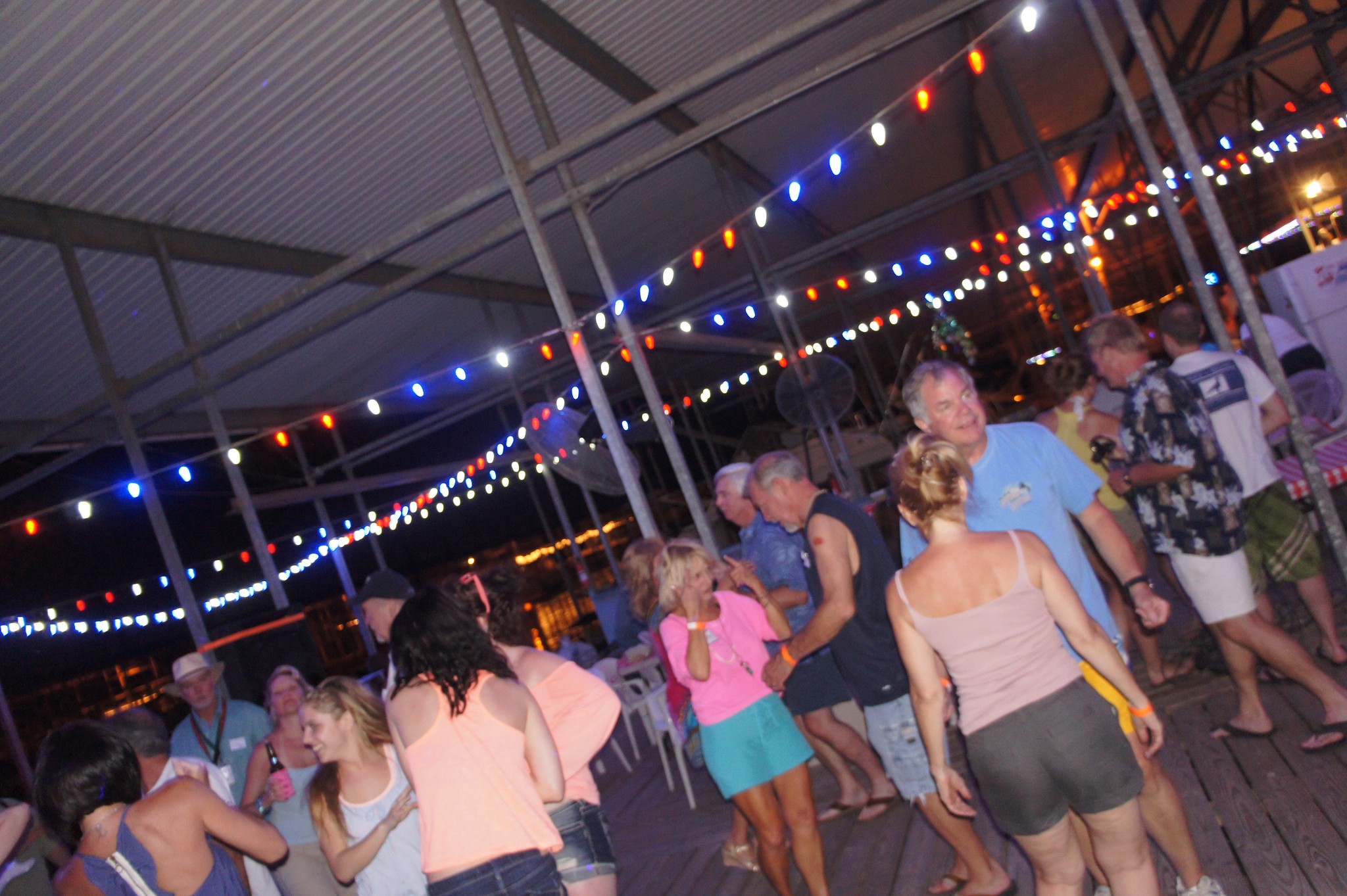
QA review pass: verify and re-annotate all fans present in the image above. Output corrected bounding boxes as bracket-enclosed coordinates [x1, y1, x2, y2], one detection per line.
[772, 353, 858, 488]
[524, 397, 642, 497]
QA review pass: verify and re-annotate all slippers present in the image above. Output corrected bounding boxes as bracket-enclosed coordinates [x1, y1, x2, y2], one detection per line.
[1256, 669, 1292, 684]
[858, 793, 900, 820]
[1209, 719, 1276, 739]
[929, 872, 966, 896]
[1301, 720, 1347, 754]
[1149, 659, 1196, 689]
[994, 877, 1019, 896]
[815, 801, 856, 822]
[1316, 643, 1347, 668]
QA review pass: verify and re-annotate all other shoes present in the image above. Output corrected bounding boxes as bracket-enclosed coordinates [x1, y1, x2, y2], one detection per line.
[1174, 872, 1224, 896]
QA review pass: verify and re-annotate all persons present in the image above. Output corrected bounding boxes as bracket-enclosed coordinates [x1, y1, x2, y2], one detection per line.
[1234, 305, 1325, 378]
[885, 434, 1164, 896]
[1158, 300, 1347, 682]
[654, 544, 829, 895]
[467, 572, 622, 896]
[0, 570, 432, 896]
[747, 451, 1019, 896]
[1077, 314, 1347, 753]
[900, 360, 1223, 896]
[1036, 356, 1196, 684]
[625, 535, 791, 871]
[386, 584, 568, 896]
[715, 462, 900, 821]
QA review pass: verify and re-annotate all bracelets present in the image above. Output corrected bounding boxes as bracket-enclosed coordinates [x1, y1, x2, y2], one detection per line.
[1124, 469, 1133, 485]
[940, 678, 951, 686]
[781, 645, 797, 666]
[686, 622, 705, 629]
[1126, 575, 1148, 590]
[1128, 702, 1156, 719]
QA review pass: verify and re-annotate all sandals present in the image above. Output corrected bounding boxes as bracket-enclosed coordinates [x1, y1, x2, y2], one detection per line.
[750, 835, 791, 856]
[720, 834, 761, 871]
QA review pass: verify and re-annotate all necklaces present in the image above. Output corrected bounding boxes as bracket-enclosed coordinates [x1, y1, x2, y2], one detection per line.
[704, 611, 754, 673]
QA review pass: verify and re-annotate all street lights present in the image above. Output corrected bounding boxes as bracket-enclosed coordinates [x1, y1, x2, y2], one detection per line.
[1304, 179, 1331, 247]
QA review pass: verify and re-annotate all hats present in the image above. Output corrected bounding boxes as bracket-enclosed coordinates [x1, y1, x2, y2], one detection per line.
[346, 568, 414, 605]
[163, 651, 224, 696]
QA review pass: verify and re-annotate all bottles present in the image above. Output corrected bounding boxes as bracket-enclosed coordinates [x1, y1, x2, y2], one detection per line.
[265, 741, 297, 799]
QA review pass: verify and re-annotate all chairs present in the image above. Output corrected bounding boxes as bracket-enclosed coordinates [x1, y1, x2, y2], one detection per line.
[575, 656, 697, 811]
